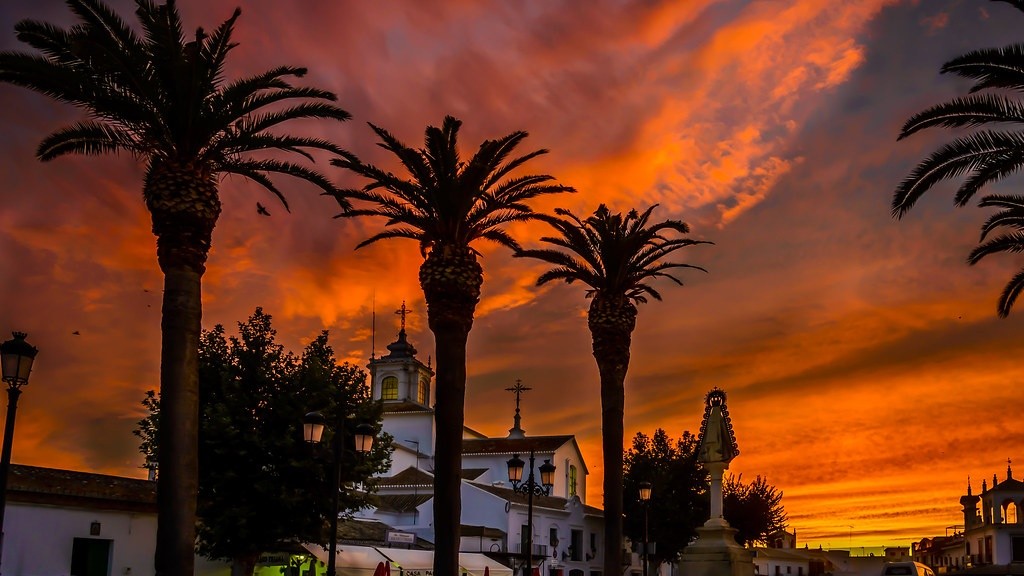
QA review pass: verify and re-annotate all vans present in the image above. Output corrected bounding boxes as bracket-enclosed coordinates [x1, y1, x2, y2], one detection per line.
[880, 560, 935, 576]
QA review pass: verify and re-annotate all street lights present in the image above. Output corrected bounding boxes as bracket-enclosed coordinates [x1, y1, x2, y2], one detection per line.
[304, 406, 376, 576]
[637, 479, 654, 576]
[404, 438, 420, 527]
[0, 329, 39, 572]
[505, 445, 557, 576]
